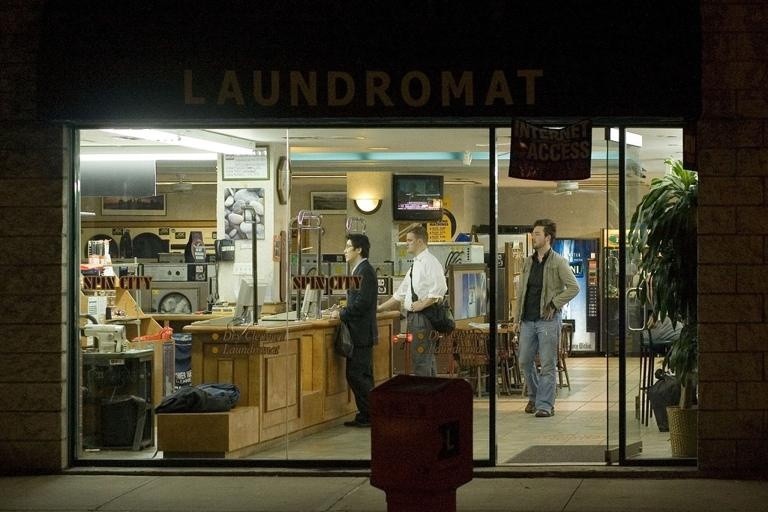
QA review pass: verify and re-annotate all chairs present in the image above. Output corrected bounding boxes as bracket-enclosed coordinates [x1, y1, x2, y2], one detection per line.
[453, 318, 576, 400]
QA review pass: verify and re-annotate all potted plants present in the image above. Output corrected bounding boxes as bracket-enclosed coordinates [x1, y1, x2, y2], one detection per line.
[629, 159, 699, 456]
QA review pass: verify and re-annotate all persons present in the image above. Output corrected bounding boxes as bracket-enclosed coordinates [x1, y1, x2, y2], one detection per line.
[376, 225, 448, 377]
[643, 310, 686, 346]
[330, 233, 380, 429]
[511, 219, 579, 418]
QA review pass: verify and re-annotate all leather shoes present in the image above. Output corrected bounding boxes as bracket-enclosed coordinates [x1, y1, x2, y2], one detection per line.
[525, 401, 536, 413]
[344, 421, 355, 427]
[535, 408, 554, 417]
[358, 422, 370, 427]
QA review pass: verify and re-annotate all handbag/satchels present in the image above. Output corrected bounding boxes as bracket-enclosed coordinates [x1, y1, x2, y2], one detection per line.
[410, 294, 455, 334]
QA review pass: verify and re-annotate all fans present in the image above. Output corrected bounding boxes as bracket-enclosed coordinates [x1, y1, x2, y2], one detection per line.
[522, 180, 609, 196]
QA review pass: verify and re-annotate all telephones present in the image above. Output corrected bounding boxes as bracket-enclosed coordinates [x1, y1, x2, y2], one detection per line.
[215, 239, 235, 262]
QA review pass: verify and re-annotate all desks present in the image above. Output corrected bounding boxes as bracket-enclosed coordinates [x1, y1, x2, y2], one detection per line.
[113, 310, 226, 339]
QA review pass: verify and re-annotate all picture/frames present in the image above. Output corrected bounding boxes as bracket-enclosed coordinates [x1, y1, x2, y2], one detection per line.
[447, 261, 488, 324]
[100, 192, 166, 216]
[309, 190, 347, 215]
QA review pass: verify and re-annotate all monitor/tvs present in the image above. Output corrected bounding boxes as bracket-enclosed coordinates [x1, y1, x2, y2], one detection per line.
[392, 174, 444, 221]
